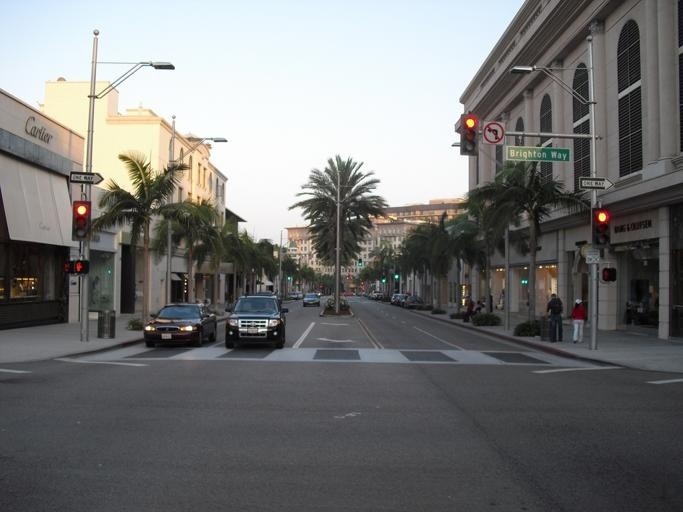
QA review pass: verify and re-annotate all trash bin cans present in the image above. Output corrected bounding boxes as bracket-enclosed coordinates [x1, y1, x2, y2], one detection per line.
[97, 309, 115, 339]
[541, 315, 554, 342]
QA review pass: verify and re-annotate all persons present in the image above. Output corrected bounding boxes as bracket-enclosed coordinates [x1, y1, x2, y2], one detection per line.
[546, 294, 563, 342]
[464, 296, 476, 322]
[626, 299, 633, 326]
[639, 292, 652, 312]
[570, 299, 587, 343]
[475, 300, 482, 313]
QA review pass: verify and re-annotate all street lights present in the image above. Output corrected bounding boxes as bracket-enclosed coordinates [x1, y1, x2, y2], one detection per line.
[510, 35, 601, 349]
[166, 115, 227, 304]
[80, 28, 175, 342]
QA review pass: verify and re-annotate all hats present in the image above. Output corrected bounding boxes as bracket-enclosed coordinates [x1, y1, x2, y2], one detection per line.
[576, 298, 582, 305]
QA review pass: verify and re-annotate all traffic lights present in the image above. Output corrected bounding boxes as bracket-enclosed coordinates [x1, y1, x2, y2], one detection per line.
[593, 209, 611, 248]
[394, 273, 400, 280]
[381, 275, 387, 284]
[358, 257, 363, 267]
[460, 113, 480, 155]
[58, 260, 90, 274]
[603, 267, 617, 282]
[72, 200, 91, 241]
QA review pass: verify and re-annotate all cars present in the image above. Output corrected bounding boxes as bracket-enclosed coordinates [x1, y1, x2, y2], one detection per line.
[368, 290, 384, 300]
[391, 294, 424, 309]
[143, 303, 217, 347]
[225, 291, 322, 349]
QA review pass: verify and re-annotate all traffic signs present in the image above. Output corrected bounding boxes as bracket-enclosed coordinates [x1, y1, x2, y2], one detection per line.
[579, 176, 615, 191]
[70, 171, 104, 184]
